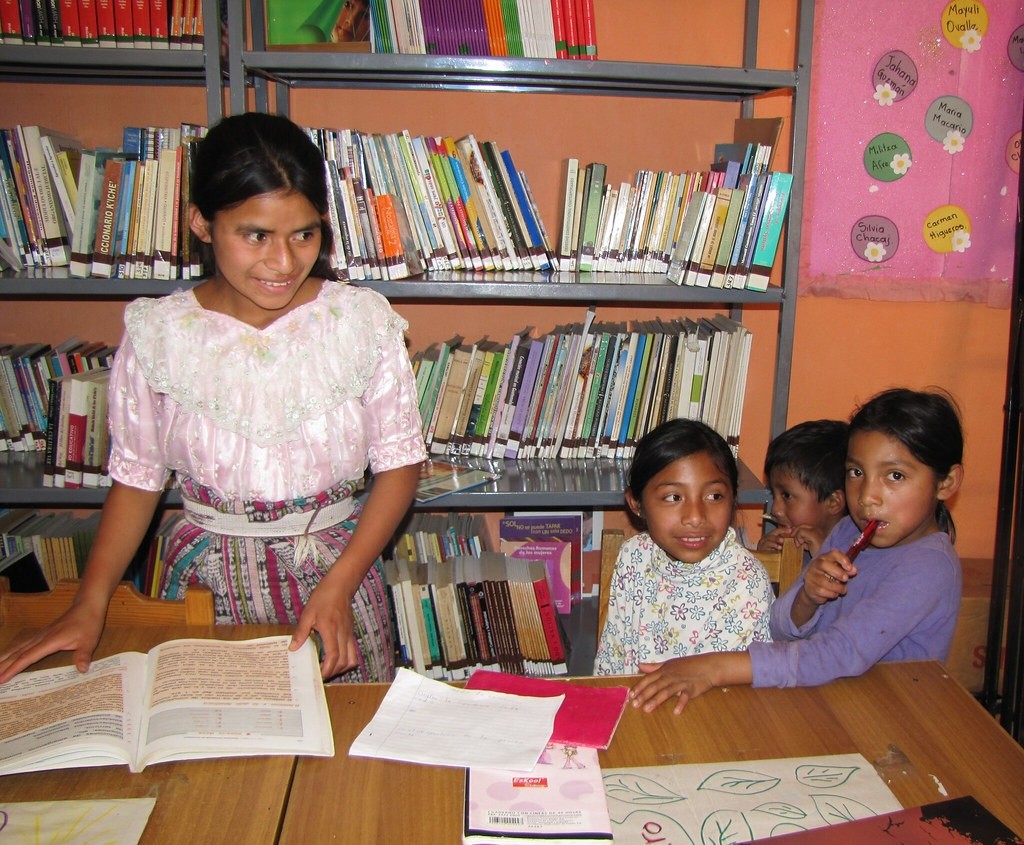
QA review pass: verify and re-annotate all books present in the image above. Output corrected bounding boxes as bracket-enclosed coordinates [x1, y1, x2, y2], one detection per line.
[464, 669, 630, 750]
[462, 742, 613, 845]
[369, 0, 599, 60]
[304, 117, 793, 681]
[0, 0, 205, 50]
[0, 123, 209, 601]
[0, 635, 335, 776]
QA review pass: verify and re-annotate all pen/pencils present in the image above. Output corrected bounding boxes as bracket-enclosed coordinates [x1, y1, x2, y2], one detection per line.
[759, 513, 779, 525]
[829, 520, 877, 582]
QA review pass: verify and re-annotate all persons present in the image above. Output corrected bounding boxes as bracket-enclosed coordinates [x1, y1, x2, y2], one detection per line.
[0, 113, 428, 685]
[756, 420, 851, 595]
[592, 418, 777, 676]
[630, 388, 962, 717]
[330, 0, 370, 42]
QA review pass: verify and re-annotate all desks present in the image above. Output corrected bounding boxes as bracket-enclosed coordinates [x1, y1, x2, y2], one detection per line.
[279, 659, 1024, 845]
[0, 625, 321, 844]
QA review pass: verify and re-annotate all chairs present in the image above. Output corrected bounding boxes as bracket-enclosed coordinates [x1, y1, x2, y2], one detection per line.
[0, 577, 216, 624]
[596, 529, 803, 648]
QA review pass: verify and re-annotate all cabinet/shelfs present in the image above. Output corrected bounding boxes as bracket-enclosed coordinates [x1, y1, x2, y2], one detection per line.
[2, 1, 222, 507]
[227, 1, 817, 675]
